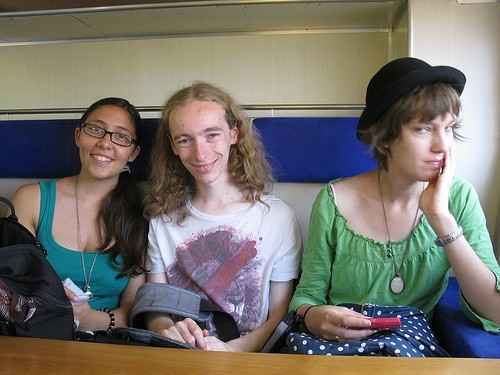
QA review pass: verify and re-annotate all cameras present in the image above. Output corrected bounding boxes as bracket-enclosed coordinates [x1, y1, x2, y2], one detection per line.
[347, 316, 402, 331]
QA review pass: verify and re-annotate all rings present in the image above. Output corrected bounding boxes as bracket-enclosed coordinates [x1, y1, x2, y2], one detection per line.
[335, 335, 339, 343]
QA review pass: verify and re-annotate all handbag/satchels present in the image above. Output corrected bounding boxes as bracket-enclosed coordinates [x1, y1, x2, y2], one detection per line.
[286, 303, 450, 358]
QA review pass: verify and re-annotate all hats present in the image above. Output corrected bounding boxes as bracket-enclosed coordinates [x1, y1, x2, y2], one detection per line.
[356, 56, 466, 144]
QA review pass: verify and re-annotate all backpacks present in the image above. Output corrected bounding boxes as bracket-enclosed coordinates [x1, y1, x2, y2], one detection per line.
[110, 282, 240, 351]
[0, 196, 74, 340]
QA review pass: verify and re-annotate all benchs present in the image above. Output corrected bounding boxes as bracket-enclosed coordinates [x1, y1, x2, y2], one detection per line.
[0, 108, 500, 359]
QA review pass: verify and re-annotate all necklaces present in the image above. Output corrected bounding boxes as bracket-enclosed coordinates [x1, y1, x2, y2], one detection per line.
[378, 166, 420, 295]
[75, 173, 100, 299]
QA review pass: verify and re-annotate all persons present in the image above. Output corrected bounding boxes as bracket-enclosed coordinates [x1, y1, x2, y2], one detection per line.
[144, 82, 302, 351]
[286, 57, 500, 356]
[7, 97, 146, 331]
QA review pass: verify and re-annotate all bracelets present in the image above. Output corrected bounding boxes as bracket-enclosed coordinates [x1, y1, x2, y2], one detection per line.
[96, 307, 115, 334]
[295, 305, 314, 336]
[434, 225, 463, 247]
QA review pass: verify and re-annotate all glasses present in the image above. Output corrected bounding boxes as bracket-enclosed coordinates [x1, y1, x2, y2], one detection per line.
[80, 123, 137, 147]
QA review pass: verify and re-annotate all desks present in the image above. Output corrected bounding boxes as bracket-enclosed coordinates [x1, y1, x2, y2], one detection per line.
[0, 336, 499, 375]
